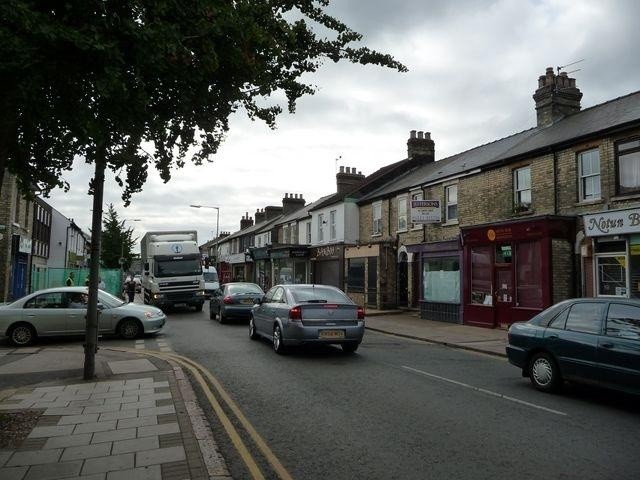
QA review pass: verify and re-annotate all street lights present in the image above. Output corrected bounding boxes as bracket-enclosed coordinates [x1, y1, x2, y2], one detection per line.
[191, 204, 219, 268]
[120, 218, 141, 268]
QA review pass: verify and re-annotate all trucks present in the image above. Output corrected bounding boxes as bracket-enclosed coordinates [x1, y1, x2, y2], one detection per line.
[140, 230, 210, 312]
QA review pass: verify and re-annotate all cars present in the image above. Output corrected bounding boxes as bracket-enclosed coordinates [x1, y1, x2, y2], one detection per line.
[0, 286, 166, 346]
[123, 276, 141, 293]
[249, 283, 366, 353]
[209, 282, 265, 323]
[503, 297, 639, 400]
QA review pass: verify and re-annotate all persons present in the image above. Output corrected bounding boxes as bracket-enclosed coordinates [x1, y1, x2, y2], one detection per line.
[98, 277, 106, 291]
[67, 272, 75, 286]
[125, 273, 136, 303]
[85, 272, 90, 286]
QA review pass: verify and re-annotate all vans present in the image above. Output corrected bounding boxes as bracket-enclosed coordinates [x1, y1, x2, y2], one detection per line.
[202, 266, 220, 297]
